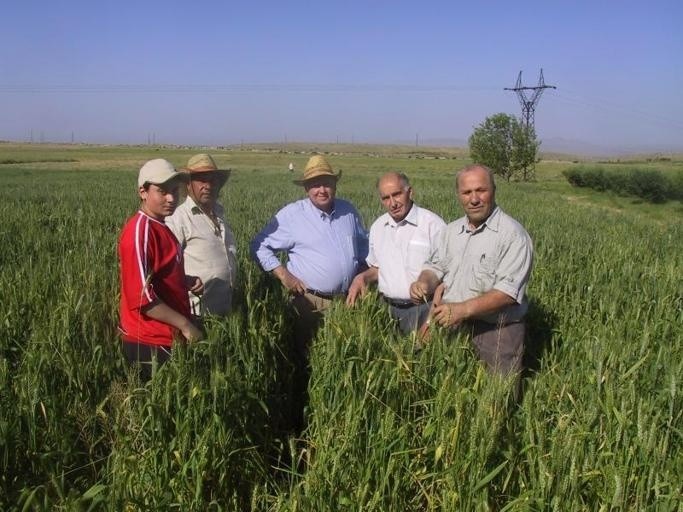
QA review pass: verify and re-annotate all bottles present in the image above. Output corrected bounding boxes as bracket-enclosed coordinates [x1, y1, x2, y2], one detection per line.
[291, 155, 343, 187]
[177, 153, 231, 184]
[137, 158, 189, 192]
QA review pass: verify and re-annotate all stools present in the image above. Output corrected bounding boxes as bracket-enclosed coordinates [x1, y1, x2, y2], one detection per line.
[306, 290, 336, 300]
[381, 295, 416, 308]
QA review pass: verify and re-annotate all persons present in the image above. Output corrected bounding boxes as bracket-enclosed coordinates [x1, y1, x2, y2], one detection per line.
[163, 152, 239, 337]
[117, 157, 204, 384]
[408, 163, 534, 401]
[248, 155, 369, 358]
[345, 171, 446, 338]
